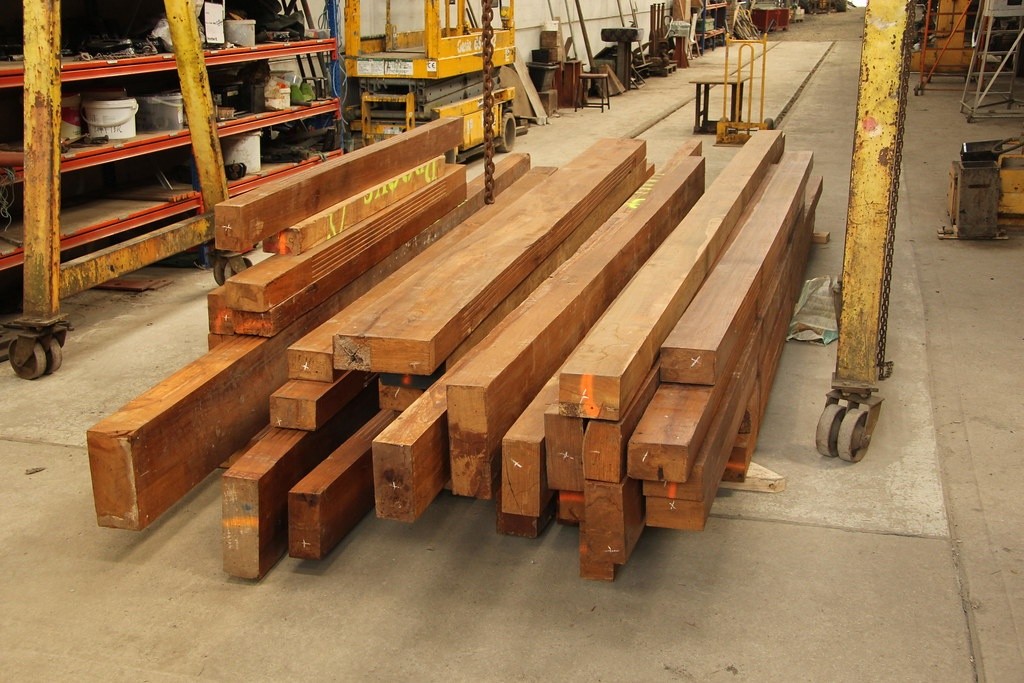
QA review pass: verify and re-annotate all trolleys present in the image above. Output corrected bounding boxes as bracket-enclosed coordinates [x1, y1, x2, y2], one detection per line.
[715, 34, 777, 148]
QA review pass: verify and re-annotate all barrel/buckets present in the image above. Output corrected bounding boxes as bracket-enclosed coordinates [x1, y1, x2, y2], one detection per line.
[82, 98, 139, 141]
[223, 19, 257, 47]
[58, 89, 82, 140]
[134, 94, 184, 132]
[266, 87, 292, 110]
[222, 130, 262, 174]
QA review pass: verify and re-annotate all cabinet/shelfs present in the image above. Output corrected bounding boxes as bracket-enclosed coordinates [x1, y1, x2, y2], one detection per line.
[690, 1, 727, 56]
[0, 27, 347, 273]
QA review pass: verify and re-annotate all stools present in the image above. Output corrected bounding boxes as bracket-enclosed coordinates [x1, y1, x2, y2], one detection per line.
[689, 73, 750, 134]
[573, 72, 611, 113]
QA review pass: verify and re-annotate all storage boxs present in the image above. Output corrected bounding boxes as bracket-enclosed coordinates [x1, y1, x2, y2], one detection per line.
[752, 8, 790, 34]
